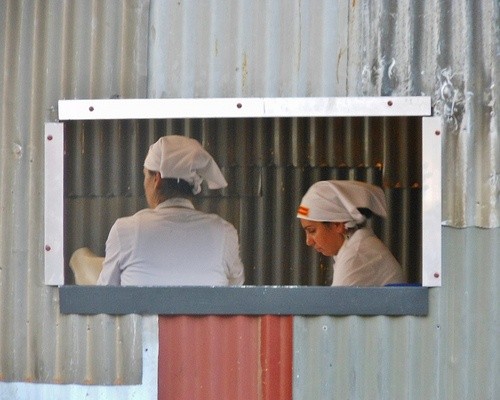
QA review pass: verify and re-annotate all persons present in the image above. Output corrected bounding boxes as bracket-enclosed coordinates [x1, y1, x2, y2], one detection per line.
[297, 179, 406, 287]
[95, 135, 245, 287]
